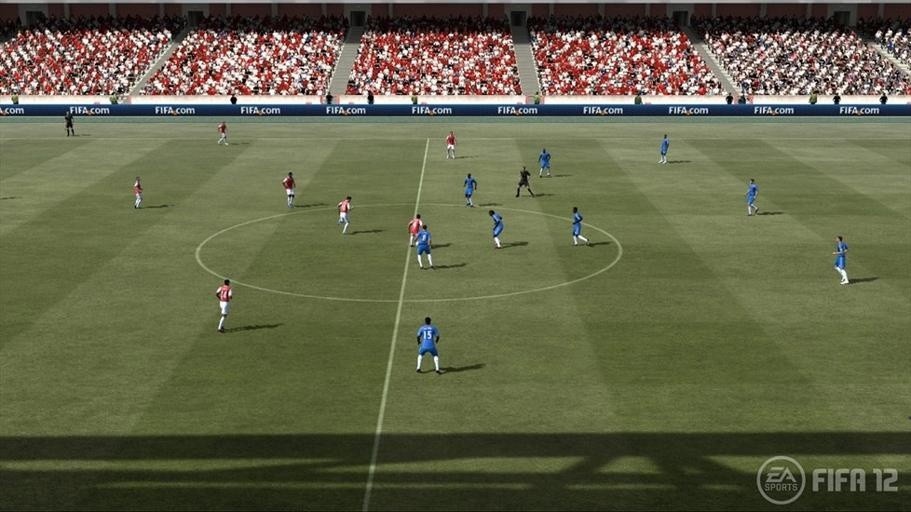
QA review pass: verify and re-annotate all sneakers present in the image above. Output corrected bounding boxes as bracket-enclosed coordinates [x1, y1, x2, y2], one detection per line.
[494, 242, 503, 249]
[409, 245, 415, 248]
[286, 202, 295, 211]
[538, 174, 552, 179]
[337, 221, 349, 235]
[657, 160, 668, 165]
[216, 141, 222, 146]
[572, 242, 591, 247]
[419, 266, 436, 271]
[465, 203, 475, 209]
[745, 208, 759, 217]
[446, 155, 456, 160]
[416, 369, 444, 375]
[839, 277, 851, 286]
[134, 204, 142, 210]
[515, 194, 536, 200]
[217, 326, 227, 334]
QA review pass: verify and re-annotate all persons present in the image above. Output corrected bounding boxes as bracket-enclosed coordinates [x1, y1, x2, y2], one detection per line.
[416, 317, 442, 374]
[65, 110, 74, 137]
[215, 279, 232, 332]
[133, 176, 143, 208]
[537, 148, 551, 177]
[282, 172, 296, 210]
[658, 134, 669, 165]
[572, 207, 589, 246]
[445, 131, 456, 159]
[463, 173, 477, 207]
[408, 214, 423, 247]
[414, 225, 433, 269]
[217, 121, 230, 146]
[489, 210, 503, 249]
[832, 235, 849, 285]
[515, 166, 535, 198]
[744, 178, 759, 216]
[337, 196, 353, 234]
